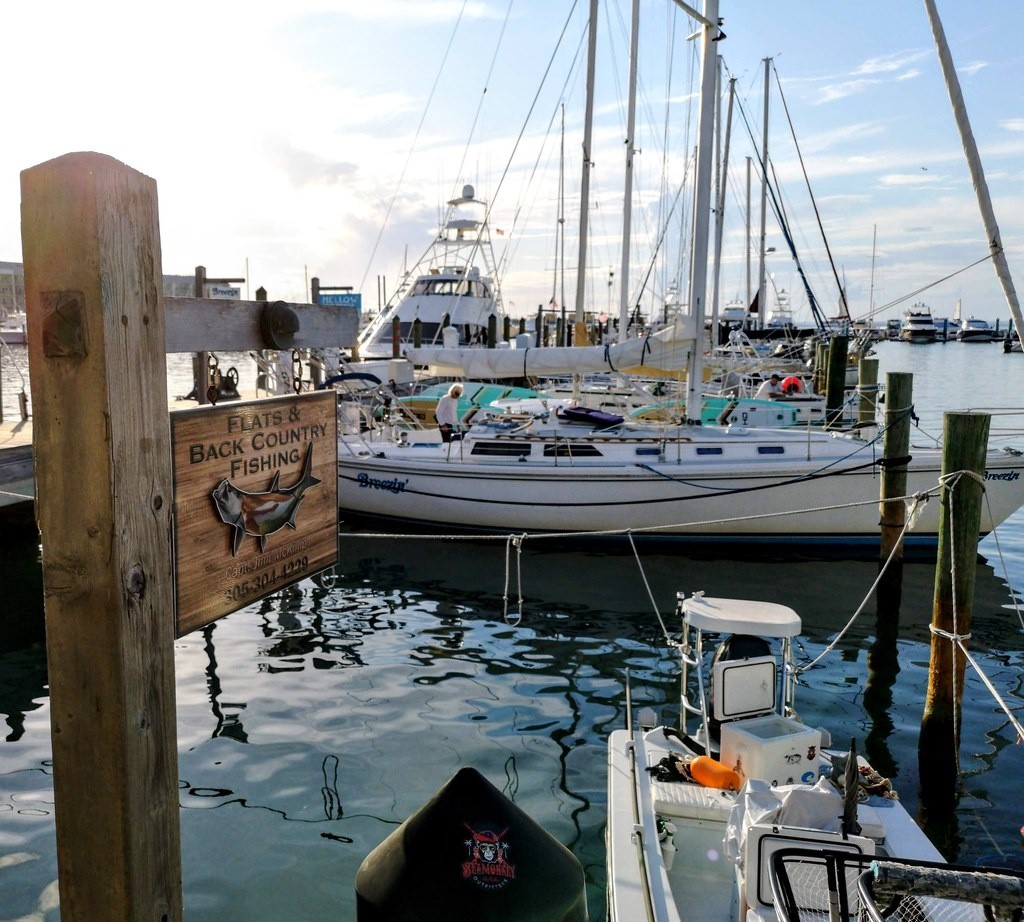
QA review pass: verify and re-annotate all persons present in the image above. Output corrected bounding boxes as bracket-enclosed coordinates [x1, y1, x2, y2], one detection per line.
[435, 383, 463, 443]
[753, 373, 788, 402]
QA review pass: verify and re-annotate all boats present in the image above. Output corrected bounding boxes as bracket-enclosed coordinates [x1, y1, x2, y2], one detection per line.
[900, 302, 938, 343]
[603, 590, 995, 922]
[956, 316, 997, 343]
[0, 313, 28, 344]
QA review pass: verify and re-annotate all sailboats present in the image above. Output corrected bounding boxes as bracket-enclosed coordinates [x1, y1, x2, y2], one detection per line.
[248, 0, 1024, 563]
